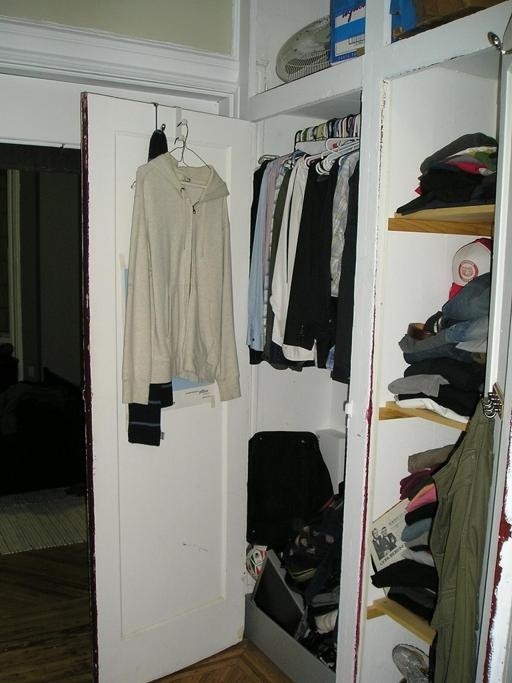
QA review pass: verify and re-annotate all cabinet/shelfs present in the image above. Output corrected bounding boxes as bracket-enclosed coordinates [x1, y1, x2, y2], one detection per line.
[81, 0, 512, 683]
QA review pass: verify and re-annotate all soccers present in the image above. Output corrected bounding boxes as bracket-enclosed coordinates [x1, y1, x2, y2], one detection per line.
[246, 542, 269, 582]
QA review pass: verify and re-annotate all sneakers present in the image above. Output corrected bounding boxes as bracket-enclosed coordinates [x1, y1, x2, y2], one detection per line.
[393, 644, 431, 683]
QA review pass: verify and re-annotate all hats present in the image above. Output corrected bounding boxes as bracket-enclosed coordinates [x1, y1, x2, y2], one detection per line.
[448, 238, 494, 299]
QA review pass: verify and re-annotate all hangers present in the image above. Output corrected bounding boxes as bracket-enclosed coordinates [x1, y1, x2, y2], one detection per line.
[131, 137, 189, 192]
[169, 120, 212, 188]
[258, 113, 358, 174]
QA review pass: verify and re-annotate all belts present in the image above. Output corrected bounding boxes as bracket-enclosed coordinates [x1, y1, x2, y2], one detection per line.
[408, 323, 433, 340]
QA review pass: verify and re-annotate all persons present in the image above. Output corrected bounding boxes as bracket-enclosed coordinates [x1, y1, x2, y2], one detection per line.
[372, 528, 390, 561]
[381, 527, 397, 551]
